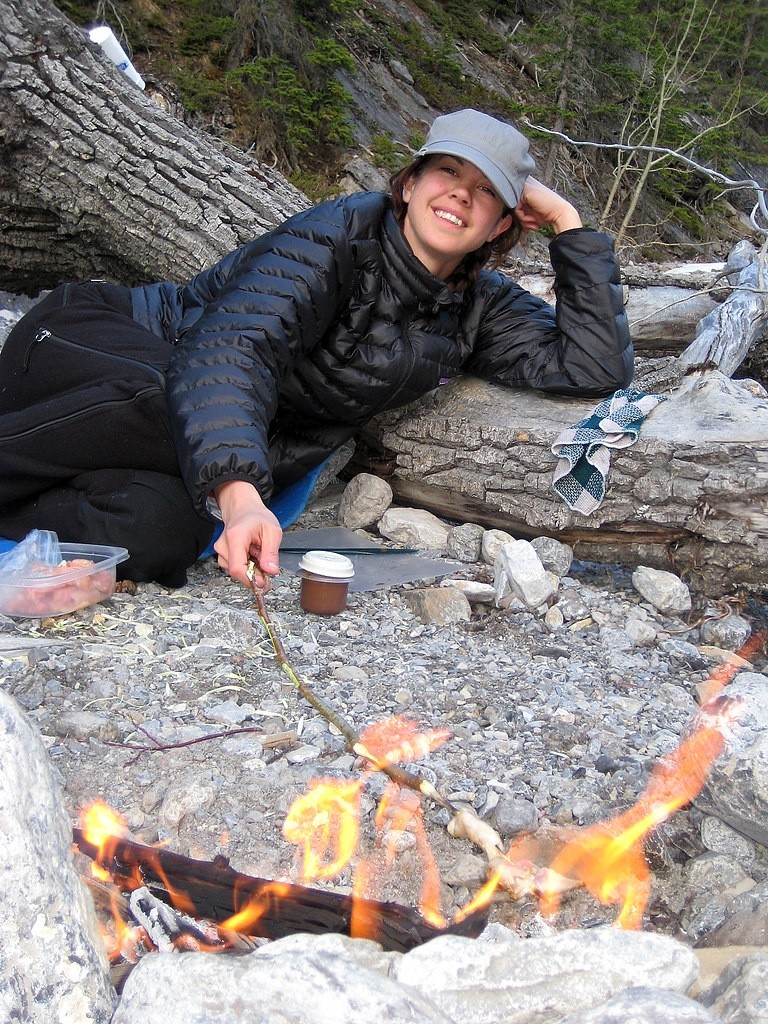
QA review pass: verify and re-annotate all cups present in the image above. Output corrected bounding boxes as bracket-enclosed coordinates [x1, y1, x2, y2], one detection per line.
[296, 569, 356, 613]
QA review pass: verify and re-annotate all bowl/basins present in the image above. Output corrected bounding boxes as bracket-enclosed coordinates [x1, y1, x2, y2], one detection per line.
[0, 541, 129, 618]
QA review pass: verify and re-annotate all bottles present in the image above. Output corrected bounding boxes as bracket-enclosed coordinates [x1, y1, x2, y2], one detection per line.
[89, 27, 147, 92]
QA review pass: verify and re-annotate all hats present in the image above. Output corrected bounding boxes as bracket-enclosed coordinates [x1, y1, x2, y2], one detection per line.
[413, 107, 535, 209]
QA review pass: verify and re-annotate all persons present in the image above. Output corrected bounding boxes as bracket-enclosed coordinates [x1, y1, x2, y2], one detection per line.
[0, 109, 635, 589]
